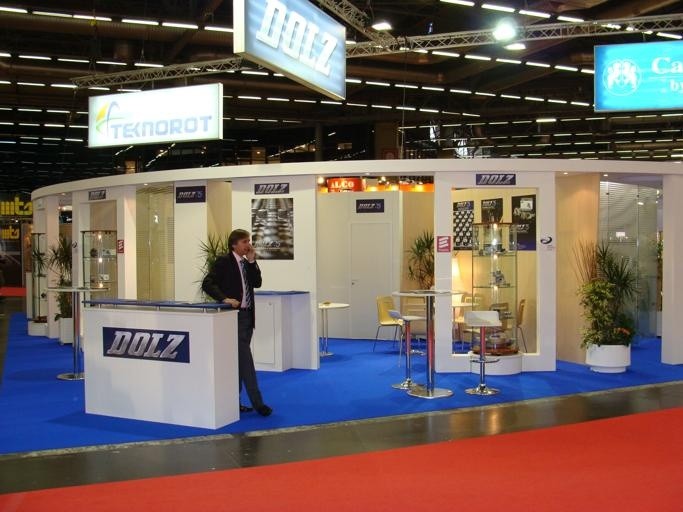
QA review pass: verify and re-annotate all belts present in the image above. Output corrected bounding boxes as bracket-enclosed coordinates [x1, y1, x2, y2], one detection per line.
[238, 307, 253, 312]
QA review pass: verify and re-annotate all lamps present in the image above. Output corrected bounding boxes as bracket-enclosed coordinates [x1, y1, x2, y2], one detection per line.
[366, 0, 393, 32]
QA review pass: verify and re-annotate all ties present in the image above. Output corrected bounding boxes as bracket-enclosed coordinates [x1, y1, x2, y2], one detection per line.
[238, 259, 252, 306]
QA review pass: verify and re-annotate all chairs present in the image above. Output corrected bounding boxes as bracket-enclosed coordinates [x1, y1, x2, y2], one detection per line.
[372, 293, 528, 397]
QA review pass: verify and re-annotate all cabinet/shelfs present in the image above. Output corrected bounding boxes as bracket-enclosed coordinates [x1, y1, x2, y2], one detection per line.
[471, 221, 518, 356]
[248, 290, 310, 373]
[28, 233, 48, 336]
[82, 230, 117, 307]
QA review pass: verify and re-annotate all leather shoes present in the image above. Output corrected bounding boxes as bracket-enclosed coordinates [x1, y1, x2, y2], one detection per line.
[253, 403, 273, 417]
[239, 404, 253, 414]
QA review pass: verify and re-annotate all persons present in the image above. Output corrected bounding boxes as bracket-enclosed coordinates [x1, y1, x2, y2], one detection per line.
[203, 229, 273, 416]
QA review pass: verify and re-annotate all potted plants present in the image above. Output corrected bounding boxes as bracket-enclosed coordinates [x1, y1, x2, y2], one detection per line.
[47, 235, 73, 346]
[569, 239, 641, 373]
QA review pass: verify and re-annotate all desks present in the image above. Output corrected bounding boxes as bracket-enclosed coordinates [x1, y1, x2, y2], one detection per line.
[44, 286, 112, 381]
[317, 302, 349, 357]
